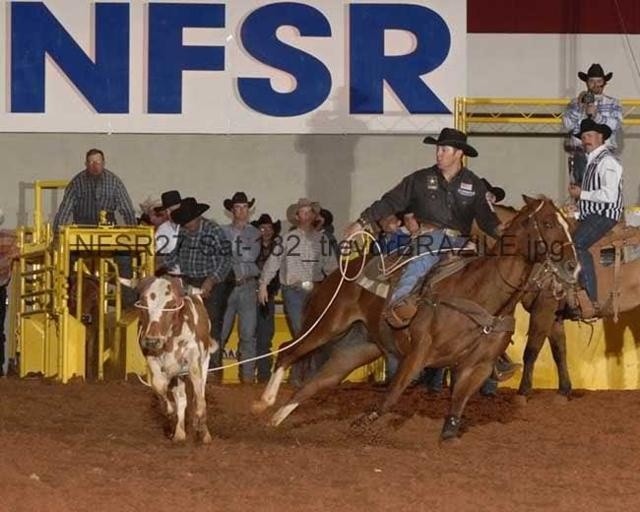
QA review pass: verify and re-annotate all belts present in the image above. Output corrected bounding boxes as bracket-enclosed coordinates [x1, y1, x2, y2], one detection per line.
[301, 281, 314, 291]
[444, 229, 462, 237]
[235, 277, 251, 286]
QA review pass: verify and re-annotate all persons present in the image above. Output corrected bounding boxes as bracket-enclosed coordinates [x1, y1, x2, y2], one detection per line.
[148, 203, 166, 225]
[424, 177, 506, 395]
[260, 197, 338, 388]
[403, 208, 419, 236]
[248, 213, 282, 384]
[53, 148, 137, 311]
[217, 191, 262, 388]
[554, 119, 622, 318]
[0, 224, 32, 376]
[153, 190, 182, 291]
[374, 214, 406, 390]
[155, 197, 233, 369]
[318, 209, 339, 262]
[343, 128, 506, 322]
[562, 63, 624, 203]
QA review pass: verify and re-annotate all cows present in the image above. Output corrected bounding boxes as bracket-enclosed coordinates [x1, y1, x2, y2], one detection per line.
[116, 275, 218, 443]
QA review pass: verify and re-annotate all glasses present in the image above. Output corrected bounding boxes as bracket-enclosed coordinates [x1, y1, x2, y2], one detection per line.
[260, 227, 272, 231]
[87, 160, 102, 165]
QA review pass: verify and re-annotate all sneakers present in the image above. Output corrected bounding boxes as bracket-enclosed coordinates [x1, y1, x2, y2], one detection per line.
[496, 356, 521, 374]
[568, 305, 600, 318]
[381, 304, 417, 322]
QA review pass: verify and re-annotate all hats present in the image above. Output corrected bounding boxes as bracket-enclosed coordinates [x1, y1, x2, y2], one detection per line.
[171, 198, 210, 224]
[224, 192, 255, 211]
[424, 129, 477, 156]
[481, 179, 505, 202]
[155, 191, 181, 210]
[579, 64, 612, 81]
[321, 209, 333, 226]
[287, 199, 319, 225]
[575, 120, 611, 139]
[251, 215, 280, 232]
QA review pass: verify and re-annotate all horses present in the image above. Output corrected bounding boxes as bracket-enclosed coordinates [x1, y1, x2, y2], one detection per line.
[249, 195, 581, 449]
[469, 203, 640, 407]
